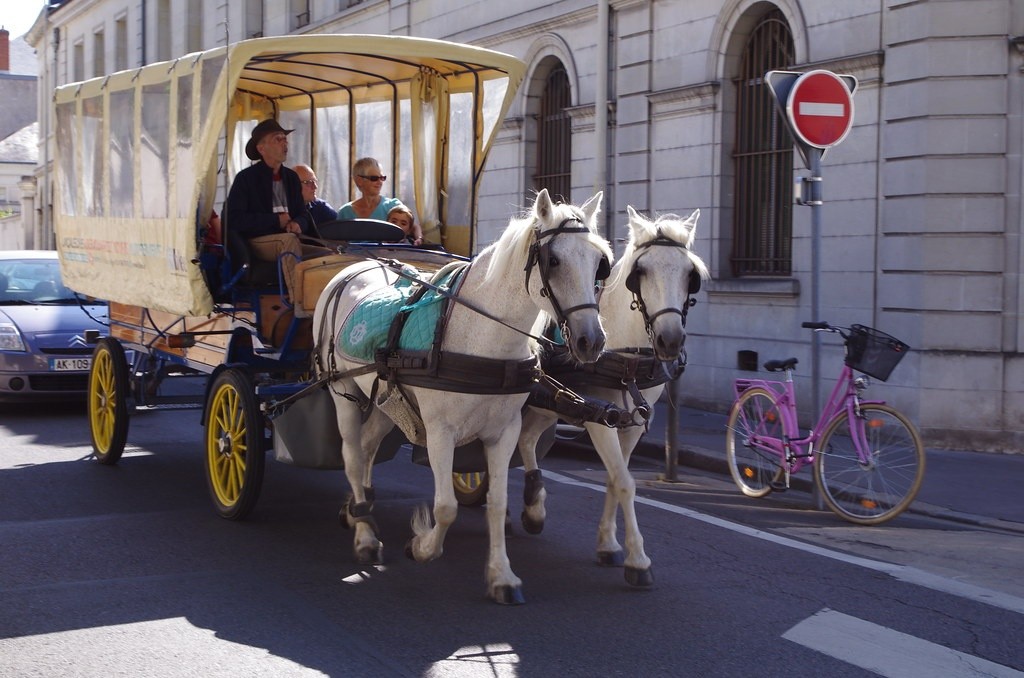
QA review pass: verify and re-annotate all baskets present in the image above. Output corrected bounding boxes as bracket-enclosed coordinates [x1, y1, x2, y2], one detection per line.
[843, 322, 909, 383]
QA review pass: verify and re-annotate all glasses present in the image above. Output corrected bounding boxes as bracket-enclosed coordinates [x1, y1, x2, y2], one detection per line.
[300, 180, 319, 186]
[358, 174, 386, 181]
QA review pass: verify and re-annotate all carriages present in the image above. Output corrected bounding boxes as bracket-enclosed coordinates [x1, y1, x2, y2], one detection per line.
[50, 32, 707, 605]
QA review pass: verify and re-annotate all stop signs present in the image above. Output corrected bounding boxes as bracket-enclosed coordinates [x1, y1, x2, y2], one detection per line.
[783, 68, 856, 149]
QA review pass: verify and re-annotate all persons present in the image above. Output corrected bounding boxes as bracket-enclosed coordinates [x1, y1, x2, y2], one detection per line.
[206, 209, 222, 244]
[291, 164, 338, 225]
[230, 119, 335, 305]
[337, 157, 424, 246]
[386, 205, 414, 234]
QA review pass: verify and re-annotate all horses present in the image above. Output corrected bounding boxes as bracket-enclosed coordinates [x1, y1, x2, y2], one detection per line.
[312, 186, 614, 607]
[481, 204, 711, 587]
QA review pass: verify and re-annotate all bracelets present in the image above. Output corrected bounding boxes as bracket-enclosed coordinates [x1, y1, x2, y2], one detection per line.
[284, 220, 292, 229]
[418, 237, 423, 240]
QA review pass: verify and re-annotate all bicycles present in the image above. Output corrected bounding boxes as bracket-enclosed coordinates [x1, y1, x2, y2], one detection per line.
[724, 318, 925, 524]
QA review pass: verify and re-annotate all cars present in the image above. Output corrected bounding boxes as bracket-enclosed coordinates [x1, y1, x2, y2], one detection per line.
[0, 247, 154, 412]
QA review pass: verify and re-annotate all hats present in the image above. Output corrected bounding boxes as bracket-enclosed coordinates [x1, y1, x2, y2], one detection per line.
[245, 119, 294, 161]
